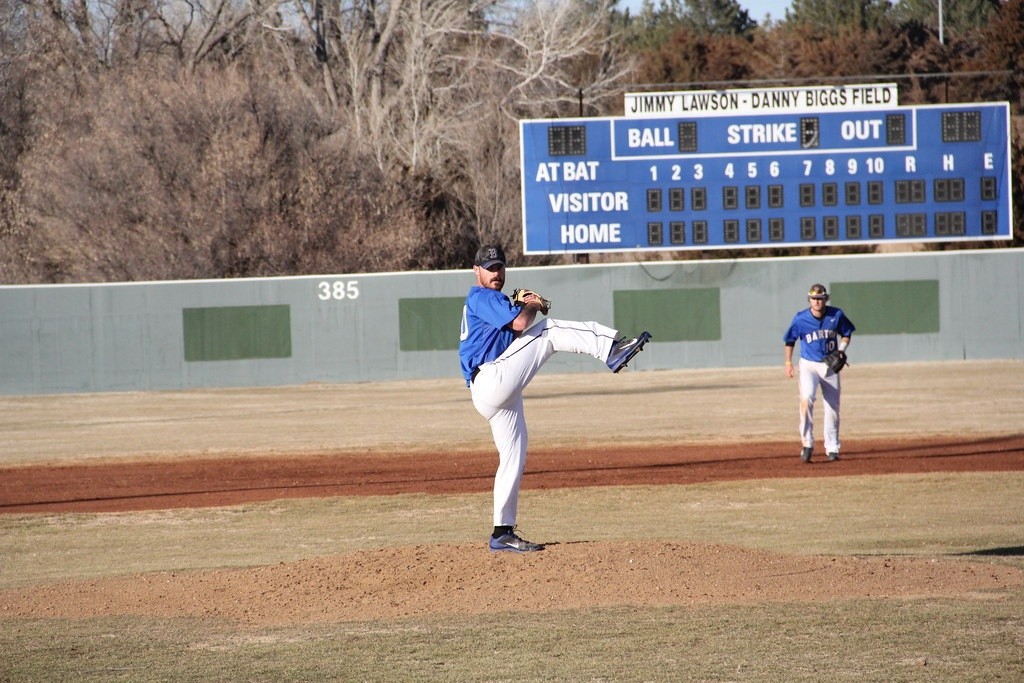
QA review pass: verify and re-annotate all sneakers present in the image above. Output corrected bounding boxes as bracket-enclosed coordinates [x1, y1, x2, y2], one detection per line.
[607, 332, 652, 373]
[801, 445, 813, 463]
[829, 453, 838, 461]
[489, 525, 542, 552]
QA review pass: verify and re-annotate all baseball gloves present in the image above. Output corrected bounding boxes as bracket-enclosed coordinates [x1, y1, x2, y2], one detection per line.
[821, 349, 847, 374]
[511, 288, 549, 315]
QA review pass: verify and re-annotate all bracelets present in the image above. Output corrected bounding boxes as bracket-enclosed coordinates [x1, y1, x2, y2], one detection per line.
[838, 342, 847, 351]
[785, 362, 791, 365]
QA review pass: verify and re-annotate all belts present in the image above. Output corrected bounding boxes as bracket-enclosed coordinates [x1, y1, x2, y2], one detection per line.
[471, 367, 481, 384]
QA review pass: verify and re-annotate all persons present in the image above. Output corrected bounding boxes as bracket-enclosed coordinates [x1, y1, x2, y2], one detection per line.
[783, 283, 856, 461]
[458, 243, 654, 553]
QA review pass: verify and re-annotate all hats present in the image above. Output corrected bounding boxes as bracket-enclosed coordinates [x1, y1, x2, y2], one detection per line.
[808, 284, 827, 299]
[474, 245, 506, 269]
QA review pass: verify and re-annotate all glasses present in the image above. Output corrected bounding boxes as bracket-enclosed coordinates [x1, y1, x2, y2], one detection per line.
[809, 289, 825, 297]
